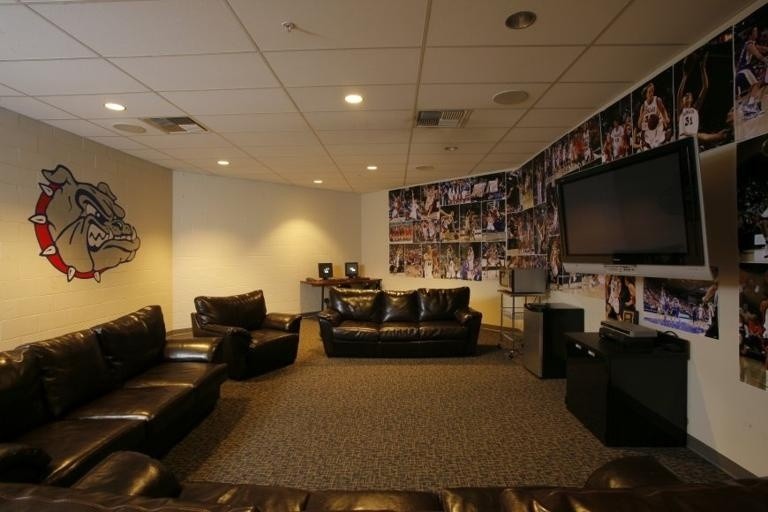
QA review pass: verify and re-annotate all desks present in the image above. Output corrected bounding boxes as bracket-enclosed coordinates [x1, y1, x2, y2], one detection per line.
[301, 277, 384, 319]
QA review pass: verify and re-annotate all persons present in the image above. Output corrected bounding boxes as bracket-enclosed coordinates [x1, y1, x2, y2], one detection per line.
[390, 24, 768, 373]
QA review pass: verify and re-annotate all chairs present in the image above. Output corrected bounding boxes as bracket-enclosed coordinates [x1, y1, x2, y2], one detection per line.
[189, 289, 303, 383]
[363, 278, 383, 290]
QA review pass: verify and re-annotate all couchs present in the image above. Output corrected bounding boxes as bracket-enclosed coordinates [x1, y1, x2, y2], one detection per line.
[0, 448, 768, 512]
[317, 283, 484, 359]
[0, 303, 230, 490]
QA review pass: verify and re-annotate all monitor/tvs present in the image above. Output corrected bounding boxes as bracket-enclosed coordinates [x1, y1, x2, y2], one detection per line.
[345, 262, 359, 278]
[319, 263, 333, 280]
[555, 135, 714, 282]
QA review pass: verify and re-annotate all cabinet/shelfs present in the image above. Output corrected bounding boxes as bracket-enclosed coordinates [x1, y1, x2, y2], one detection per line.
[559, 330, 690, 450]
[497, 286, 544, 359]
[522, 301, 585, 380]
